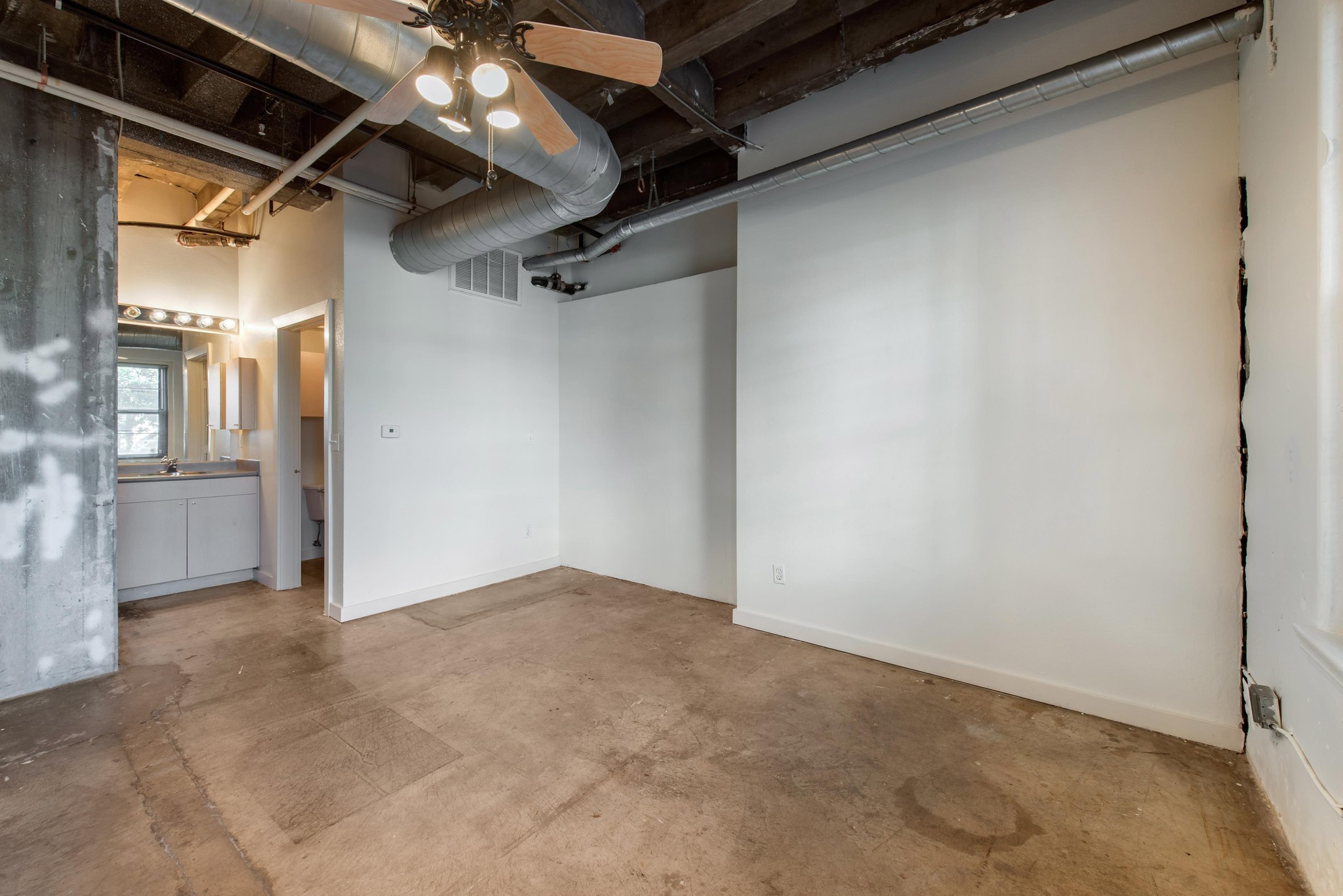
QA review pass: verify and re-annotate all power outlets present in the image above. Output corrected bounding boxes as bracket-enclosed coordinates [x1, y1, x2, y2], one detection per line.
[331, 434, 340, 452]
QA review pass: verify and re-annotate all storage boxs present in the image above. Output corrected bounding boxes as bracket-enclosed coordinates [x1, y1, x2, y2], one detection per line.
[208, 362, 227, 430]
[225, 357, 258, 430]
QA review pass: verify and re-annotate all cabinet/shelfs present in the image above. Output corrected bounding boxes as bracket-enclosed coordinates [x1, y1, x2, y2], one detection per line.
[300, 351, 325, 418]
[116, 476, 260, 604]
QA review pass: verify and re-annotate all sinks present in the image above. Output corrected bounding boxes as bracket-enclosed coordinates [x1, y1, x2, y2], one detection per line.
[137, 472, 206, 483]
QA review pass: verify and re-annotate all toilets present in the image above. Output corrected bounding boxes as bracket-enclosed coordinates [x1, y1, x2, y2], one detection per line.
[302, 483, 324, 521]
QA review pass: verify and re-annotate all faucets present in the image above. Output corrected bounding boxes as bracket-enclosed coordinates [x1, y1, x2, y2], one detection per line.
[159, 456, 169, 463]
[159, 457, 183, 473]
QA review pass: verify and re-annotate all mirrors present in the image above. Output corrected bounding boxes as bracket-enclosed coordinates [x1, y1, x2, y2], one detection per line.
[116, 318, 240, 465]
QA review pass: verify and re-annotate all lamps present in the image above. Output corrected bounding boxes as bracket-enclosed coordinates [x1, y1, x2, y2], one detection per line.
[302, 0, 663, 192]
